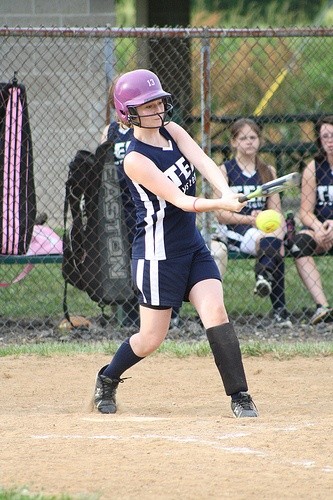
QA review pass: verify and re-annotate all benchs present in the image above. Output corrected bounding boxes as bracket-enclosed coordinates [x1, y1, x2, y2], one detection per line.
[0, 250, 333, 265]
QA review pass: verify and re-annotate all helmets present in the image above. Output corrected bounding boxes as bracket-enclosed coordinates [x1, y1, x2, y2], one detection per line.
[113, 69, 173, 128]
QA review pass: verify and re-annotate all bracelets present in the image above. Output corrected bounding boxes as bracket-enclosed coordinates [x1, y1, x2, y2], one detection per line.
[193, 197, 204, 212]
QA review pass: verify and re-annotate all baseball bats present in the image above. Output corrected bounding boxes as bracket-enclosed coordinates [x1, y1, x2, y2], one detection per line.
[238, 170, 303, 204]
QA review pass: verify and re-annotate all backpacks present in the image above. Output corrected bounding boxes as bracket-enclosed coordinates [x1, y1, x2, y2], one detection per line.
[61, 140, 138, 304]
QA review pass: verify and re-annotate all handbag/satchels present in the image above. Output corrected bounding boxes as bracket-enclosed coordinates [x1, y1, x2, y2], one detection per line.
[0, 82, 37, 254]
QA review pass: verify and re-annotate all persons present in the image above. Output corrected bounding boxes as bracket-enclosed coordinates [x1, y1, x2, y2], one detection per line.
[93, 69, 300, 416]
[211, 119, 293, 328]
[291, 116, 333, 326]
[101, 75, 187, 335]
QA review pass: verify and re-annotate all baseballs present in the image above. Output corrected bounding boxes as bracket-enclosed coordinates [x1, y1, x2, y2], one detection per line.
[256, 209, 283, 233]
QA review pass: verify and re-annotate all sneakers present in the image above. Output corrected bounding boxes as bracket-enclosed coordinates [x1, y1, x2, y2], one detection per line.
[273, 310, 291, 328]
[230, 392, 258, 417]
[253, 274, 273, 298]
[94, 364, 119, 414]
[300, 304, 333, 325]
